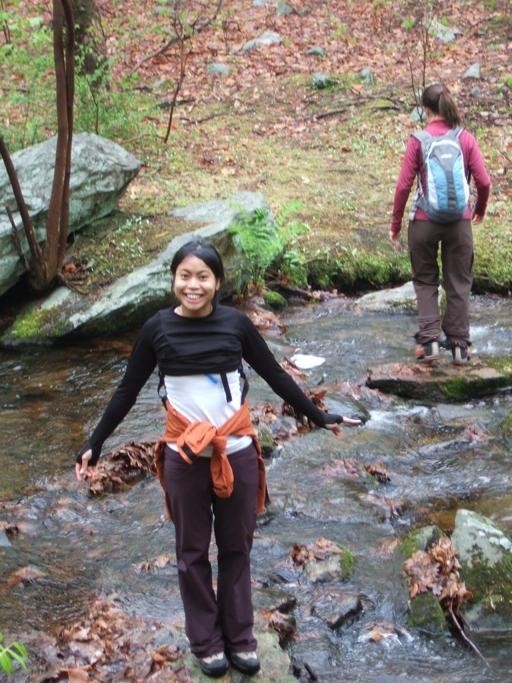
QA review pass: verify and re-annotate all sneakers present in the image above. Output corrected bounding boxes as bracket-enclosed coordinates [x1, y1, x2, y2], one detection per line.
[451, 345, 471, 365]
[416, 341, 439, 363]
[230, 651, 259, 674]
[198, 650, 229, 677]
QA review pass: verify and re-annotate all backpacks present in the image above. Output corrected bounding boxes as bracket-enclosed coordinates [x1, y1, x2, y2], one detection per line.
[412, 125, 471, 224]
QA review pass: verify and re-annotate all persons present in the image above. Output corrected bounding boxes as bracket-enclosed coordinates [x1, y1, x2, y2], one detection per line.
[388, 84, 490, 366]
[75, 239, 362, 678]
